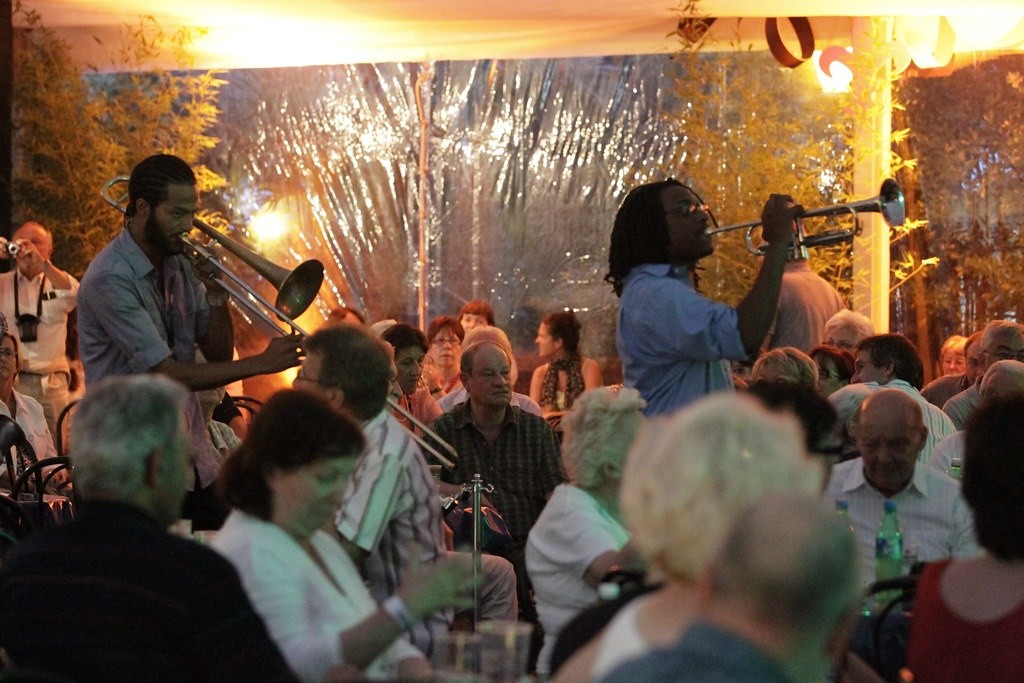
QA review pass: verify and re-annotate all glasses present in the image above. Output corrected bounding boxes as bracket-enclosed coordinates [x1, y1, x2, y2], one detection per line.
[822, 338, 859, 351]
[818, 369, 844, 382]
[978, 388, 998, 402]
[0, 349, 17, 358]
[663, 203, 709, 217]
[432, 336, 460, 346]
[295, 369, 348, 399]
[982, 349, 1024, 363]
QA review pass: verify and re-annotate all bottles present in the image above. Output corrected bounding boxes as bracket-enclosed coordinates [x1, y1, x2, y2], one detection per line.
[874, 500, 905, 612]
[946, 458, 963, 480]
[836, 499, 871, 616]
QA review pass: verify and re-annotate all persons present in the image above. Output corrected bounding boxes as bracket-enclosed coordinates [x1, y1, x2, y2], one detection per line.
[0, 221, 1023, 683]
[605, 177, 797, 412]
[76, 153, 305, 386]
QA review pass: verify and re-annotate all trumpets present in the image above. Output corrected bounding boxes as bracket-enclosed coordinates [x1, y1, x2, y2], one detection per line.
[705, 178, 906, 260]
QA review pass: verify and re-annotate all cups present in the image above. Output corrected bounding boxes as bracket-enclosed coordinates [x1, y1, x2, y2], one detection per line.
[475, 619, 533, 683]
[433, 632, 480, 683]
[428, 465, 441, 491]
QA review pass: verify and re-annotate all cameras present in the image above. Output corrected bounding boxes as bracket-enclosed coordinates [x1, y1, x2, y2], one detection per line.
[5, 241, 23, 255]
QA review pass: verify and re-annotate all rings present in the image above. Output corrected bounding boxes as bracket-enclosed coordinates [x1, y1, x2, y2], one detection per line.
[429, 371, 432, 374]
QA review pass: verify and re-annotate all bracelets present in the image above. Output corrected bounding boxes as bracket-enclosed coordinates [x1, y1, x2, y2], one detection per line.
[430, 386, 443, 395]
[43, 260, 52, 273]
[384, 594, 415, 632]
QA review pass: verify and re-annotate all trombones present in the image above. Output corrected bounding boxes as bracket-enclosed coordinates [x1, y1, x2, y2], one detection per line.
[100, 175, 460, 469]
